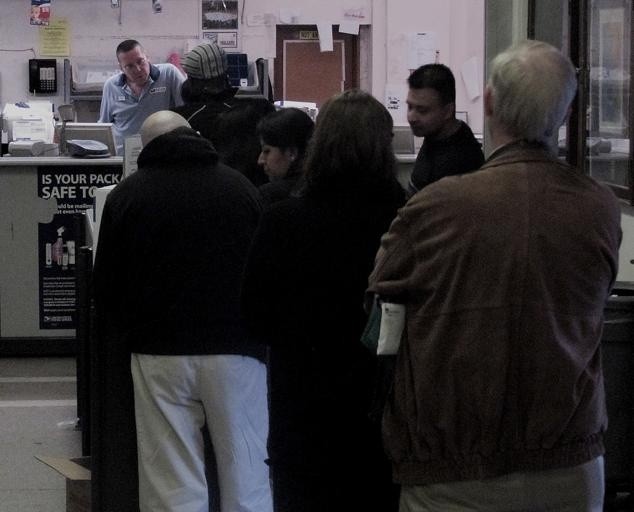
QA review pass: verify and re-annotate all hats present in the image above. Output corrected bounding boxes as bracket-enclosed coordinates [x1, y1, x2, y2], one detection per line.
[180, 43, 230, 80]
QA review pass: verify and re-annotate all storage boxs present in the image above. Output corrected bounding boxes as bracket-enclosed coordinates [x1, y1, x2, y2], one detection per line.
[34, 451, 94, 512]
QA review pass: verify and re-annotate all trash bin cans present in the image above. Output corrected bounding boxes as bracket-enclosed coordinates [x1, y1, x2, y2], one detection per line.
[598, 282, 634, 497]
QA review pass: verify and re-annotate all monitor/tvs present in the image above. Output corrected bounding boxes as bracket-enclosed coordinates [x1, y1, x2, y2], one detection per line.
[56, 122, 118, 156]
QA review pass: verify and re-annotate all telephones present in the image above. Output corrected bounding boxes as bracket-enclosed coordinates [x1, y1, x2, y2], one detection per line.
[29, 59, 57, 94]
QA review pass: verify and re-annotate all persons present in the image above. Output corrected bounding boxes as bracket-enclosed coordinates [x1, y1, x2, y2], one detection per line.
[355, 38, 624, 511]
[245, 88, 408, 510]
[94, 39, 186, 155]
[404, 63, 486, 205]
[248, 108, 315, 198]
[172, 41, 272, 141]
[69, 110, 276, 511]
[211, 98, 277, 189]
